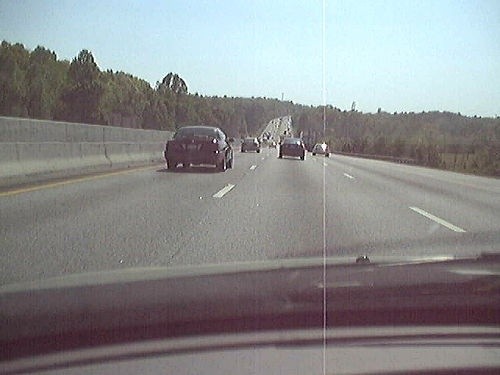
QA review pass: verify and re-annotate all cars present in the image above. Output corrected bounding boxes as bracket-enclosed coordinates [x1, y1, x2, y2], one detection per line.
[312, 143, 329, 156]
[165, 126, 234, 172]
[241, 137, 260, 153]
[269, 142, 276, 149]
[278, 138, 305, 160]
